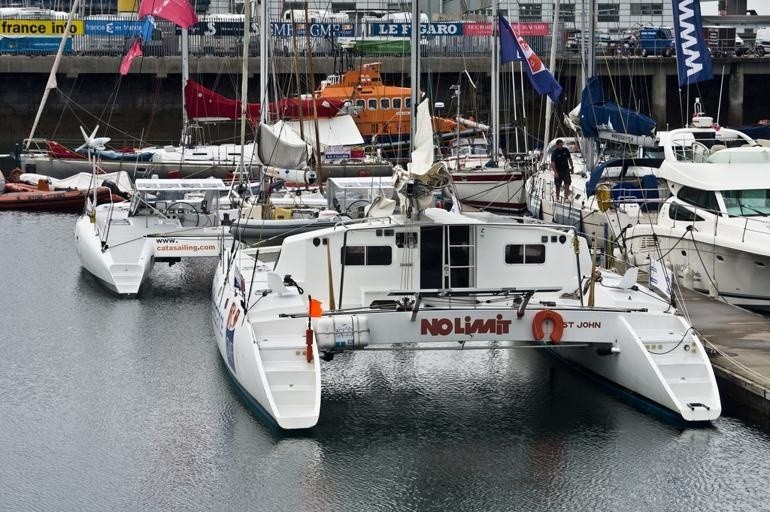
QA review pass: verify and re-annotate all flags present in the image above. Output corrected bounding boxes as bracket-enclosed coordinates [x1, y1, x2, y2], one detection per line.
[137, 1, 197, 30]
[120, 41, 145, 76]
[498, 13, 562, 102]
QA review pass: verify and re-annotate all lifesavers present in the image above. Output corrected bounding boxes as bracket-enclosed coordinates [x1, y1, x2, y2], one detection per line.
[10, 167, 23, 183]
[597, 184, 612, 211]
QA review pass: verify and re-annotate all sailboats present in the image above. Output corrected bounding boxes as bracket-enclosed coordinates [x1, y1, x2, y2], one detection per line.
[211, 0, 723, 431]
[0, 0, 770, 312]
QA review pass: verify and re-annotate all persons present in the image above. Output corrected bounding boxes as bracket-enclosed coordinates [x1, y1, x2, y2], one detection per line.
[0, 170, 6, 193]
[550, 139, 574, 202]
